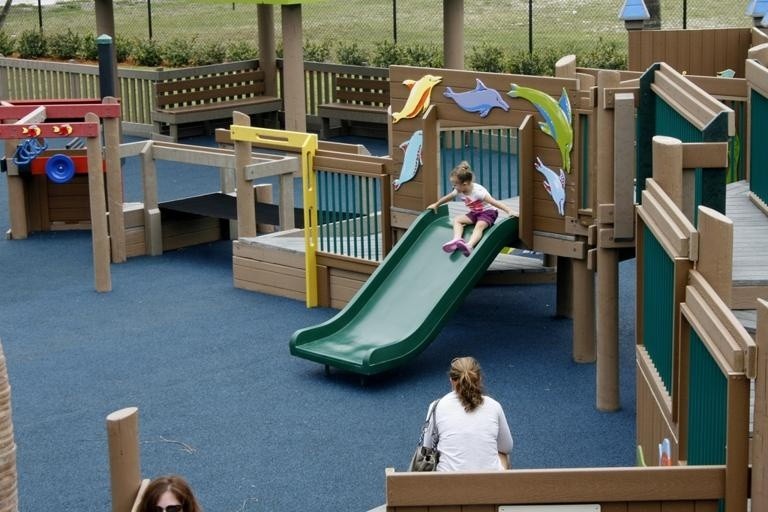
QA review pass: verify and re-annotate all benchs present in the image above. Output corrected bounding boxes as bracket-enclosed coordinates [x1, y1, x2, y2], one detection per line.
[152, 73, 388, 144]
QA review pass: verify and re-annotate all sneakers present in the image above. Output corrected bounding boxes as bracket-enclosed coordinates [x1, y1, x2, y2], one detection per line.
[442, 239, 471, 256]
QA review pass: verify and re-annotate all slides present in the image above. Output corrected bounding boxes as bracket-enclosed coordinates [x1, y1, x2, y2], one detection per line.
[289, 204, 517, 374]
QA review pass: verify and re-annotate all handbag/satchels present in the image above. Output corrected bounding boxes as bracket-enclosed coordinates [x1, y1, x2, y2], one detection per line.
[412, 398, 440, 471]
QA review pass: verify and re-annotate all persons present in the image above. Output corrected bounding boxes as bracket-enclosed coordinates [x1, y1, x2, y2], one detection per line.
[136, 478, 200, 512]
[423, 357, 513, 472]
[427, 163, 519, 257]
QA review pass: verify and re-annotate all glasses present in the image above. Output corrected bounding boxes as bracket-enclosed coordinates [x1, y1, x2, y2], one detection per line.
[149, 505, 182, 512]
